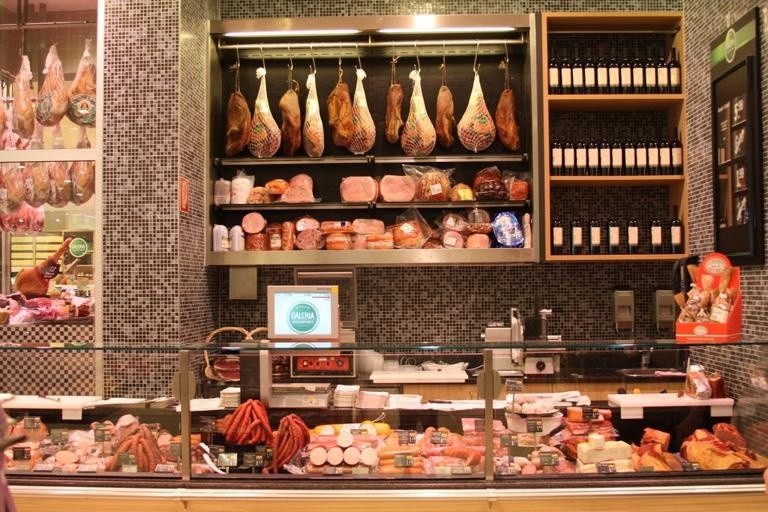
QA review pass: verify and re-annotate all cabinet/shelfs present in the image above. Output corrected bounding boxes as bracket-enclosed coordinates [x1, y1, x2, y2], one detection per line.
[209, 13, 539, 263]
[541, 11, 690, 259]
[0, 340, 768, 512]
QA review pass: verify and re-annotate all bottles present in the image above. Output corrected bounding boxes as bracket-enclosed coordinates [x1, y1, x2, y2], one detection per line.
[552, 204, 682, 254]
[552, 127, 683, 175]
[549, 44, 681, 95]
[214, 347, 240, 381]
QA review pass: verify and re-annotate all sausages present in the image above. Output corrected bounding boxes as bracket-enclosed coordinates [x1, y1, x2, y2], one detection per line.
[104, 425, 167, 472]
[222, 399, 312, 472]
[377, 441, 425, 474]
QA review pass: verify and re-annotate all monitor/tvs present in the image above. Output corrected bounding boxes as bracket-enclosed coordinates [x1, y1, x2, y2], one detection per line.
[266, 285, 340, 356]
